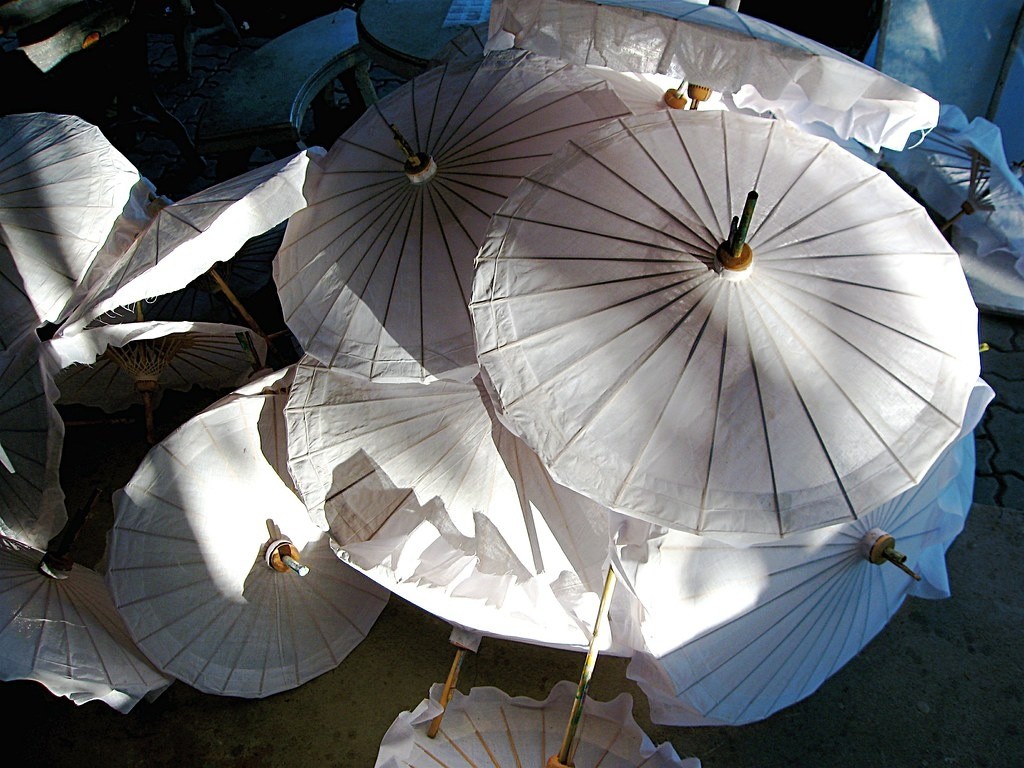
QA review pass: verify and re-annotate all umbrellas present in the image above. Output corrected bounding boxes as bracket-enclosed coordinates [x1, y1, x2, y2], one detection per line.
[1, 1, 1023, 768]
